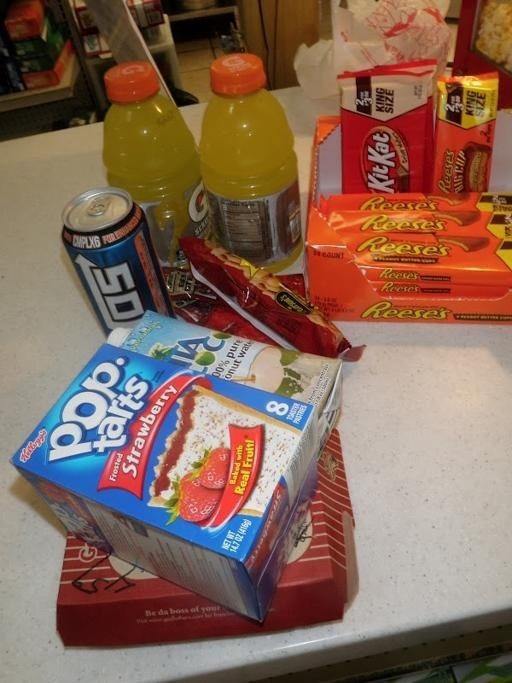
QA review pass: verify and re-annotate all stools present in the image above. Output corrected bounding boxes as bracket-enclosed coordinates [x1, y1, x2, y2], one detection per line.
[87, 13, 183, 111]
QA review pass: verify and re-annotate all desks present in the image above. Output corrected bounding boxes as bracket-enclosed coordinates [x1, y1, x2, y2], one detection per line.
[0, 84, 511, 683]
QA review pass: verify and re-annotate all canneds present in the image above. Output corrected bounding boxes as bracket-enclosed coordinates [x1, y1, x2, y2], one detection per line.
[58, 186, 177, 344]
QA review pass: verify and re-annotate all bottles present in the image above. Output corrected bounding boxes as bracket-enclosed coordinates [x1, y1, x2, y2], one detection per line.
[102, 60, 219, 269]
[197, 52, 303, 277]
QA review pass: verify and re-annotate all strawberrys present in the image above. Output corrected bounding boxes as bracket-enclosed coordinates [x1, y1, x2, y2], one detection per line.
[171, 447, 232, 524]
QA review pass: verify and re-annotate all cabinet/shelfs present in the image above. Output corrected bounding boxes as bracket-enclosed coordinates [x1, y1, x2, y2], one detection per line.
[0, 0, 100, 137]
